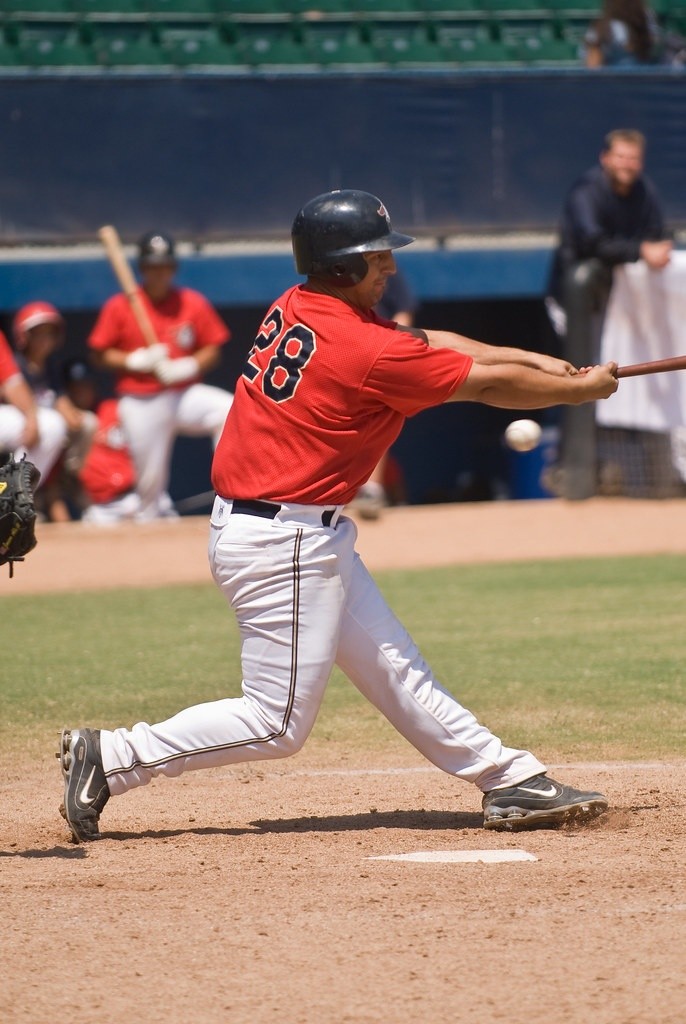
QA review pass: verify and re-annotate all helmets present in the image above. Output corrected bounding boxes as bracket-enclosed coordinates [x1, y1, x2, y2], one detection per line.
[290, 189, 416, 288]
[11, 302, 65, 350]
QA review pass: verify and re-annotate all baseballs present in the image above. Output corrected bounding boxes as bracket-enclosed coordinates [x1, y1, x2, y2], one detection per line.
[504, 419, 543, 451]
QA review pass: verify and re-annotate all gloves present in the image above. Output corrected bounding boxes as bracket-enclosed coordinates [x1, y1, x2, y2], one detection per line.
[123, 342, 168, 373]
[153, 356, 199, 386]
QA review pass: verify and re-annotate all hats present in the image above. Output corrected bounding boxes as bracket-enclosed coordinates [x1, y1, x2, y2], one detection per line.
[138, 230, 176, 266]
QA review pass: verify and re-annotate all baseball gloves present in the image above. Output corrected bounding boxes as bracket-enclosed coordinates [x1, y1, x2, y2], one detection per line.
[0, 451, 43, 577]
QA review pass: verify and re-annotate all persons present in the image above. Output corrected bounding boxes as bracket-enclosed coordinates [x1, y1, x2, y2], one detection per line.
[53, 189, 618, 841]
[0, 0, 685, 520]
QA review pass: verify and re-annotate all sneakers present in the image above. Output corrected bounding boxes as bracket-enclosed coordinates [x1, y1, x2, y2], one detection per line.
[56, 727, 110, 844]
[481, 774, 609, 831]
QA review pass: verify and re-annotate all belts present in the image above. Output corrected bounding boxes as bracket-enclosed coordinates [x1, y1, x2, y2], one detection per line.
[229, 499, 335, 526]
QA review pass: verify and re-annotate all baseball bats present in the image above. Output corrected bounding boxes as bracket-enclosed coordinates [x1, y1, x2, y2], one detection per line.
[100, 224, 173, 385]
[570, 356, 686, 381]
